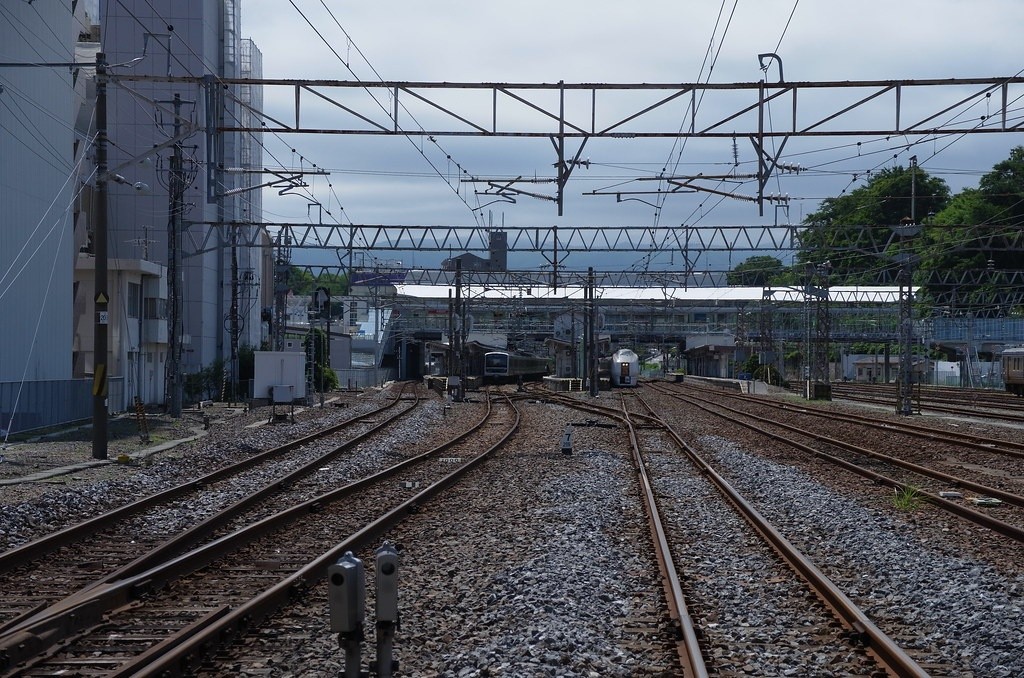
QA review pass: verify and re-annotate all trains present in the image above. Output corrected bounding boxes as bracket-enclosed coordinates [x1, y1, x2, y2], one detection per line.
[611, 349, 638, 386]
[1002, 343, 1024, 397]
[483, 351, 553, 384]
